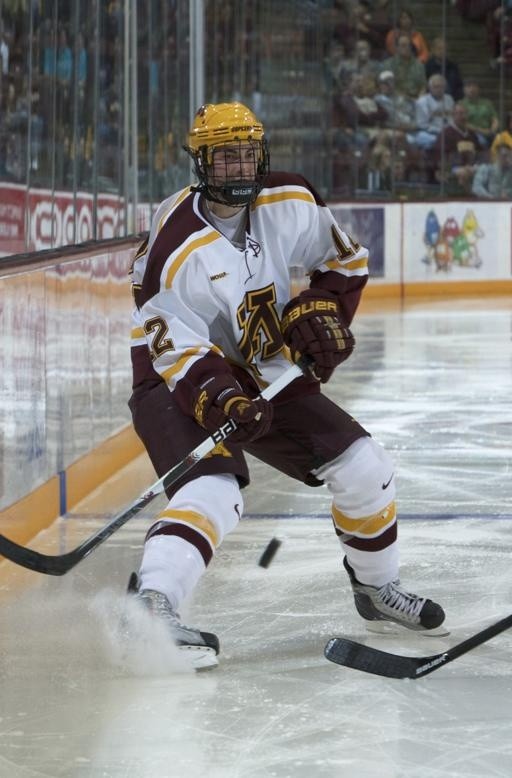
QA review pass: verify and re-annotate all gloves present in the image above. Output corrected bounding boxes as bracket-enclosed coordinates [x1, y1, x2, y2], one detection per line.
[184, 375, 272, 448]
[279, 289, 355, 383]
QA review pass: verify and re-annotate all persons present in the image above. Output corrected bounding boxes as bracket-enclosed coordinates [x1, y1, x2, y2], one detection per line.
[113, 102, 447, 654]
[1, 0, 512, 200]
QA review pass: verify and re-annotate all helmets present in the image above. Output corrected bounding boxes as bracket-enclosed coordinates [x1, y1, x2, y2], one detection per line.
[183, 101, 271, 207]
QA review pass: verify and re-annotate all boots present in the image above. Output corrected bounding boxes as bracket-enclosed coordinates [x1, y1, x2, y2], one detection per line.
[344, 556, 445, 628]
[119, 573, 220, 653]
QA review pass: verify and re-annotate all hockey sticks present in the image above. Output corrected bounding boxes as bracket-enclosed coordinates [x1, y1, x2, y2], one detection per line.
[0, 348, 310, 575]
[323, 614, 512, 683]
